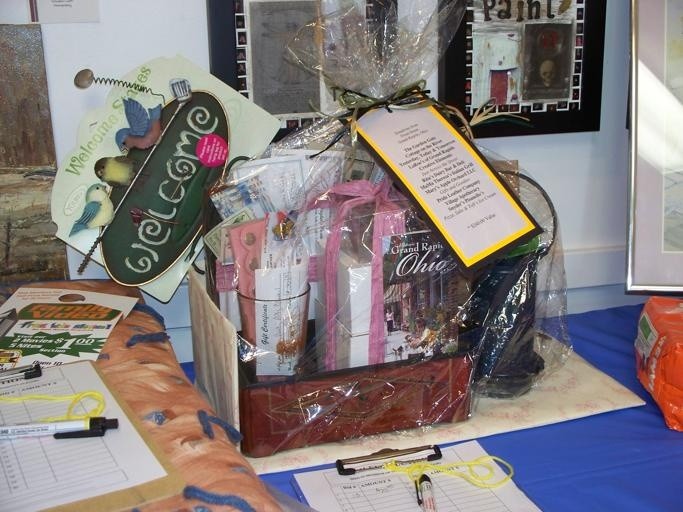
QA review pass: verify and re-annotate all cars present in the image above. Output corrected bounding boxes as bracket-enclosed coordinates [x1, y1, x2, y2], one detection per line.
[236, 283, 313, 382]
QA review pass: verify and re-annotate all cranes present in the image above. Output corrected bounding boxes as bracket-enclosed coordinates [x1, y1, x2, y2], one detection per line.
[289, 437, 545, 512]
[2, 358, 187, 512]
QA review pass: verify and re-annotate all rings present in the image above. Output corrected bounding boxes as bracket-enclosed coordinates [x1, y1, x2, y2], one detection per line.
[2, 416, 117, 441]
[414, 472, 438, 511]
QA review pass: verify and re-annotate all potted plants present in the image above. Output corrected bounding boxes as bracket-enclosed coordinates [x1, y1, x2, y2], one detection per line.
[437, 0, 607, 141]
[206, 2, 397, 144]
[626, 1, 683, 295]
